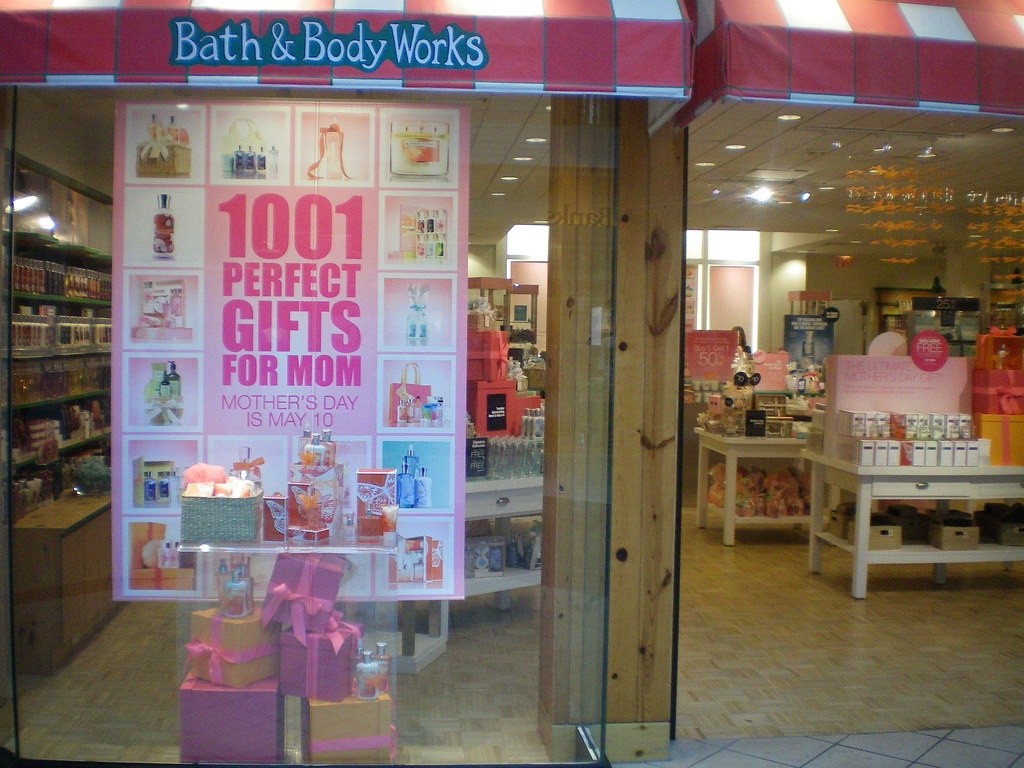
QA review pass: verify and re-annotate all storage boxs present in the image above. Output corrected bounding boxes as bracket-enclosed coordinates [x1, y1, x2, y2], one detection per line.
[160, 460, 406, 764]
[446, 600, 484, 630]
[466, 313, 548, 439]
[829, 327, 1023, 554]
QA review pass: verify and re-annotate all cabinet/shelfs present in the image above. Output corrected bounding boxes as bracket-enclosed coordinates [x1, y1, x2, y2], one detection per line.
[0, 230, 114, 681]
[872, 287, 929, 347]
[911, 296, 982, 356]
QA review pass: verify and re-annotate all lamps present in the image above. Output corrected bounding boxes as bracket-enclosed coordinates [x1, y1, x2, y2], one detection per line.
[927, 275, 947, 295]
[3, 171, 38, 216]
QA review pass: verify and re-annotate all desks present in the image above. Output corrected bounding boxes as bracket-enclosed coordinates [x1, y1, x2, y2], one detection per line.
[800, 449, 1024, 600]
[692, 427, 839, 547]
[429, 473, 542, 640]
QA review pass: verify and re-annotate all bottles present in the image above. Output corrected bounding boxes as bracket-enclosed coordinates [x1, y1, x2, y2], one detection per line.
[10, 255, 112, 453]
